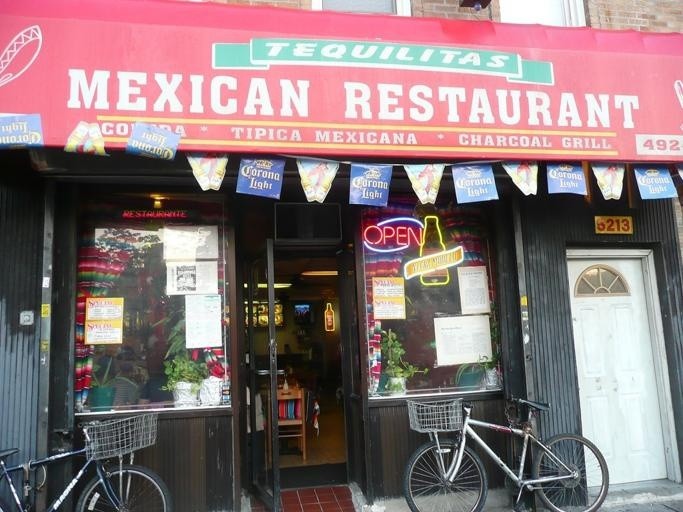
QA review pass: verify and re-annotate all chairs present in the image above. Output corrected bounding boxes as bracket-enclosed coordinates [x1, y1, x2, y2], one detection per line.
[266, 387, 307, 465]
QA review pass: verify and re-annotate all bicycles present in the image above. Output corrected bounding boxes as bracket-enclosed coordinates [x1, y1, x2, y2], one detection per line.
[0, 410, 173, 512]
[403, 395, 609, 512]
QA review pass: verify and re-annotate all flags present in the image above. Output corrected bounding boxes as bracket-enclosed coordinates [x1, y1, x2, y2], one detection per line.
[123, 122, 180, 166]
[235, 151, 288, 200]
[545, 160, 588, 196]
[451, 163, 500, 205]
[631, 163, 679, 200]
[0, 115, 45, 152]
[347, 162, 393, 207]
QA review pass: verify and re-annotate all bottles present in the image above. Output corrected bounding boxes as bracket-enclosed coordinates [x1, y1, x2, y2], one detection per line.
[502, 163, 531, 196]
[403, 164, 445, 205]
[323, 300, 336, 332]
[185, 152, 228, 193]
[590, 165, 626, 199]
[281, 379, 290, 394]
[297, 161, 340, 202]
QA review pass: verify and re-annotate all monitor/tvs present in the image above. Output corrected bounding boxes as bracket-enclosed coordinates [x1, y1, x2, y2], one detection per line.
[294, 303, 311, 325]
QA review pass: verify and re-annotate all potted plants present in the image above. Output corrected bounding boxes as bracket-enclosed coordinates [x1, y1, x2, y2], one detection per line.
[161, 354, 208, 407]
[380, 328, 428, 396]
[455, 350, 501, 390]
[88, 356, 137, 410]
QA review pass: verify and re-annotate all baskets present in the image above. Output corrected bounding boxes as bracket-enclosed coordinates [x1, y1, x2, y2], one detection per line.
[408, 396, 464, 433]
[81, 410, 157, 461]
